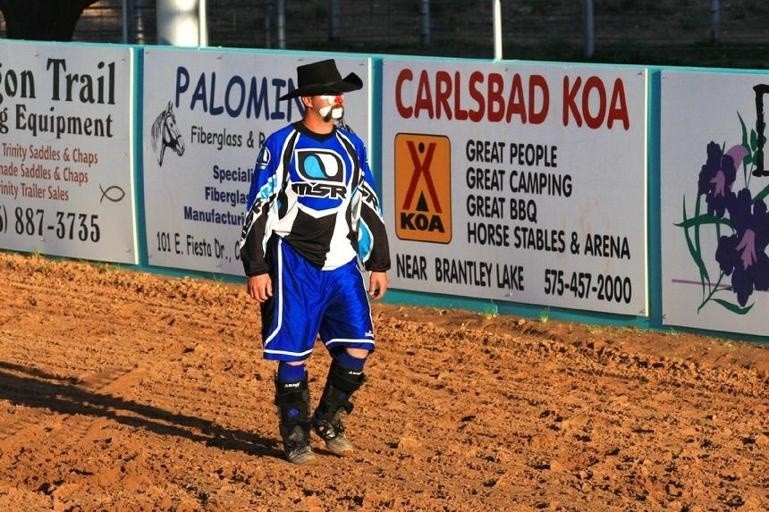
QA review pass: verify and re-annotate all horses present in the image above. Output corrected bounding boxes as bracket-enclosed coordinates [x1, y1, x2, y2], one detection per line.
[150, 100, 185, 167]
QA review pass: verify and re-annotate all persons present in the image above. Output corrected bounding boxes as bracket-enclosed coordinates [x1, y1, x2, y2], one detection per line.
[237, 59, 392, 464]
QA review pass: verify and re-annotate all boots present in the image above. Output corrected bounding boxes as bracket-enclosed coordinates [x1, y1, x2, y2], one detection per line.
[275, 376, 315, 463]
[313, 365, 367, 456]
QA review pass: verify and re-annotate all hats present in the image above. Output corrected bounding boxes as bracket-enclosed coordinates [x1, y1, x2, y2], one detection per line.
[278, 59, 364, 101]
